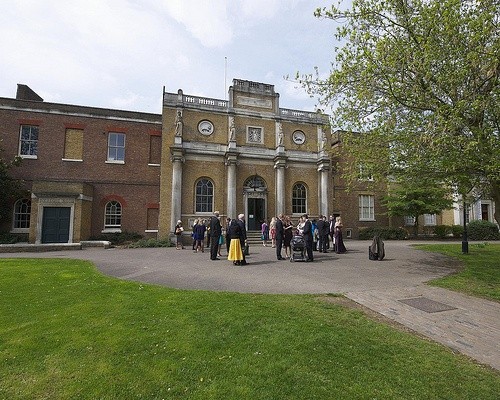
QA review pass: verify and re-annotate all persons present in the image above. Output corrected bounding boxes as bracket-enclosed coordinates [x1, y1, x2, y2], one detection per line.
[174, 220, 186, 250]
[229, 117, 237, 141]
[321, 132, 329, 156]
[262, 220, 268, 247]
[278, 127, 284, 144]
[175, 111, 184, 136]
[191, 211, 251, 266]
[269, 214, 347, 262]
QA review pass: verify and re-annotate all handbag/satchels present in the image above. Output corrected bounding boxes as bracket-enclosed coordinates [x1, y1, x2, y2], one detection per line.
[176, 228, 181, 233]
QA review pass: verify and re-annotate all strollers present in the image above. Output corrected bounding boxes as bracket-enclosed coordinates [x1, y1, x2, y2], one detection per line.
[290, 235, 308, 262]
